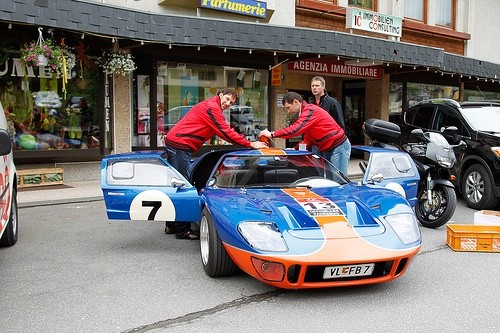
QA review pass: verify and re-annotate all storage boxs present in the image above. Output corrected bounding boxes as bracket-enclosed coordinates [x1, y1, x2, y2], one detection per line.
[362, 118, 401, 143]
[445, 209, 500, 253]
[16, 168, 64, 189]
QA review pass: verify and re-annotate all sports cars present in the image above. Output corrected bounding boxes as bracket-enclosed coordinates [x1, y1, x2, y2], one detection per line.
[100, 146, 421, 293]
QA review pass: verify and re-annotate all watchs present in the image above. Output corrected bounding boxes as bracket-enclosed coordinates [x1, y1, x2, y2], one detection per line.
[271, 131, 274, 138]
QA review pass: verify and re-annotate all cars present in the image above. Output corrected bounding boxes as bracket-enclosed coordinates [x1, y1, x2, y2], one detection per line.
[35, 90, 62, 109]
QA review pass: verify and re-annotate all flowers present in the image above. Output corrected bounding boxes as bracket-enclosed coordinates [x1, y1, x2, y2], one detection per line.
[94, 48, 137, 77]
[18, 37, 72, 80]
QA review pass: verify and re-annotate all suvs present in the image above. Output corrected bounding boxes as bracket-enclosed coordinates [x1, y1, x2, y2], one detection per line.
[398, 97, 500, 211]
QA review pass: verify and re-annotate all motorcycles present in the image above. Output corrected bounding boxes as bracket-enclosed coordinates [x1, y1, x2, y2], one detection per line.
[359, 117, 468, 228]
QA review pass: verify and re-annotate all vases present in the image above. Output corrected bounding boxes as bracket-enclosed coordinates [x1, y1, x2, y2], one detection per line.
[35, 55, 49, 67]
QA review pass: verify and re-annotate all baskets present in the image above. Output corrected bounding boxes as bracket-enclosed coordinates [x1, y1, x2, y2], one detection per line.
[446, 224, 500, 253]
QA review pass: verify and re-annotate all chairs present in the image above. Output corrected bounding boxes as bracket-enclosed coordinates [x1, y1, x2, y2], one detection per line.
[217, 167, 300, 183]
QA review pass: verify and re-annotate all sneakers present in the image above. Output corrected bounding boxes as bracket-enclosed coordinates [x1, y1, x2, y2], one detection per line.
[175, 230, 200, 240]
[165, 223, 191, 234]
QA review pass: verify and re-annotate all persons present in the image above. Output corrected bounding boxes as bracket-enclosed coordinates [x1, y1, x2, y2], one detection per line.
[164, 87, 269, 239]
[311, 76, 345, 177]
[257, 92, 351, 183]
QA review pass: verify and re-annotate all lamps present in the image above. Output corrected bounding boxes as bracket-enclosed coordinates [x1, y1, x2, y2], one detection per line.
[237, 68, 246, 80]
[253, 70, 261, 81]
